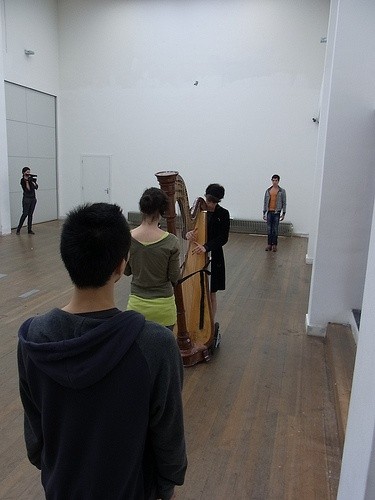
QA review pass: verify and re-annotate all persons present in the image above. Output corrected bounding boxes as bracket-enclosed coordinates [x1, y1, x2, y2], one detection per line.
[182, 183, 230, 320]
[17, 202, 187, 500]
[16, 167, 38, 235]
[123, 186, 180, 332]
[262, 174, 286, 252]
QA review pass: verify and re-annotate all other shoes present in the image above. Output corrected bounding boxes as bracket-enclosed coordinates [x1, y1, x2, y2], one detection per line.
[16, 231, 20, 235]
[265, 245, 272, 251]
[272, 245, 277, 252]
[27, 230, 35, 234]
